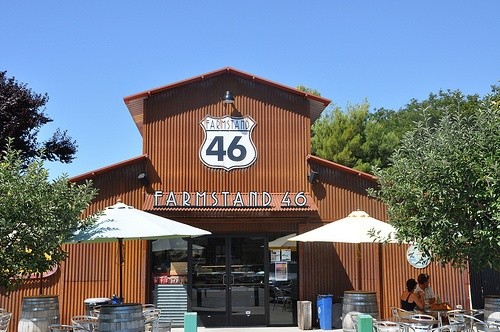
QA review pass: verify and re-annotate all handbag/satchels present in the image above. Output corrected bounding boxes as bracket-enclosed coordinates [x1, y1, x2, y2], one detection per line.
[431, 302, 452, 311]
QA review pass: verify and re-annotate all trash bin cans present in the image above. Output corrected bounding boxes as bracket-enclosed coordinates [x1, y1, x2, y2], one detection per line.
[316, 293, 333, 329]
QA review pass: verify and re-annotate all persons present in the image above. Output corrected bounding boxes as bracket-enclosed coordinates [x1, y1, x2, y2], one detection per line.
[398, 273, 450, 332]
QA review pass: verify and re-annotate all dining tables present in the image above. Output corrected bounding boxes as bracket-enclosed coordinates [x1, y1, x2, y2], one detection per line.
[425, 309, 468, 326]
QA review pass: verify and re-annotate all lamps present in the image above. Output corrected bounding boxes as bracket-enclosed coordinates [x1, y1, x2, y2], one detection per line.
[138, 173, 149, 186]
[308, 172, 319, 183]
[223, 90, 234, 103]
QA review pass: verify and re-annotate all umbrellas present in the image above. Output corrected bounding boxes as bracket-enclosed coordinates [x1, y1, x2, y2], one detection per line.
[286, 209, 430, 293]
[57, 199, 212, 304]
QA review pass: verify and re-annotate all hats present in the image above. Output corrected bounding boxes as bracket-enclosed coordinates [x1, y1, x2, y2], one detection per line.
[418, 273, 430, 283]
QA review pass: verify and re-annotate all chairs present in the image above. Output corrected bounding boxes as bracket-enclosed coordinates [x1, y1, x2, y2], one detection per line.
[48, 304, 161, 332]
[273, 287, 292, 312]
[343, 307, 500, 332]
